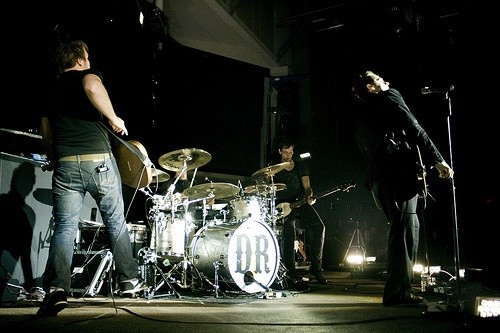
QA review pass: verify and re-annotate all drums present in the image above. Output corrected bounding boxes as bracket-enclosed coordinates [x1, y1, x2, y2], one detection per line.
[230, 196, 268, 222]
[188, 218, 281, 295]
[149, 214, 195, 264]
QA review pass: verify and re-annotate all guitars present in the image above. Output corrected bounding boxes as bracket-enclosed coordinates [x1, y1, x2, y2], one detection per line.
[41, 139, 153, 189]
[275, 179, 356, 218]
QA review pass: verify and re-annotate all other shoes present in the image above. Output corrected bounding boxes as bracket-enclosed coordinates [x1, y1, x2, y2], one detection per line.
[289, 274, 297, 285]
[309, 268, 328, 284]
[383, 293, 424, 305]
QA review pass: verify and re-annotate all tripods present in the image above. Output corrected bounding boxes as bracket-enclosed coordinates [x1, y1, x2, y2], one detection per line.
[140, 160, 229, 300]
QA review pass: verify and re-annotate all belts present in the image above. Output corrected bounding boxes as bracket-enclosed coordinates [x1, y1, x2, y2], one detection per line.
[57, 153, 114, 162]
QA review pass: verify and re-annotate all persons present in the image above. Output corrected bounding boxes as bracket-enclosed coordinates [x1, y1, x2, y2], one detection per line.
[354, 71, 455, 304]
[35, 41, 149, 318]
[266, 139, 326, 292]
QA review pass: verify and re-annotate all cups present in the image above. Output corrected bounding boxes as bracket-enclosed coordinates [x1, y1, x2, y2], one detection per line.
[421, 273, 428, 292]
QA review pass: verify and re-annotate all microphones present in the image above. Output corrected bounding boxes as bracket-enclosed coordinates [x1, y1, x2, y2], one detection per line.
[421, 84, 454, 94]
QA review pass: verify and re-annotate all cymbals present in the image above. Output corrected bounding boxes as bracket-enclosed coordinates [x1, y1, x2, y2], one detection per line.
[158, 149, 212, 171]
[243, 183, 286, 194]
[251, 162, 290, 177]
[182, 183, 240, 199]
[151, 168, 170, 183]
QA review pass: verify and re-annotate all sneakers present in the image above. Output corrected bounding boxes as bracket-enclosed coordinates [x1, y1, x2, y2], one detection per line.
[36, 286, 68, 317]
[119, 277, 149, 294]
